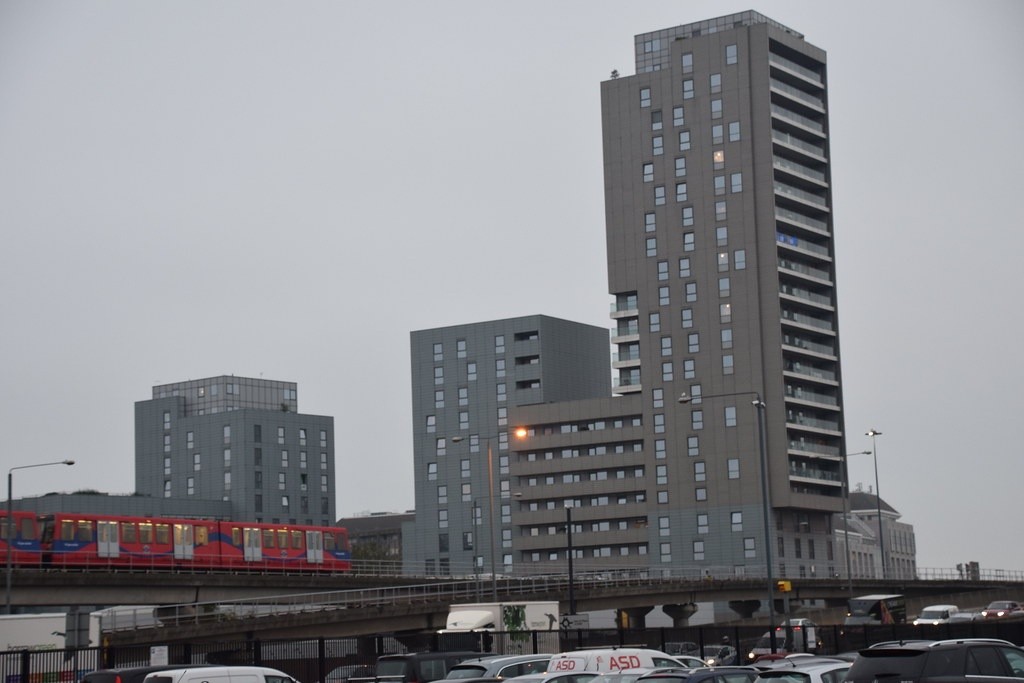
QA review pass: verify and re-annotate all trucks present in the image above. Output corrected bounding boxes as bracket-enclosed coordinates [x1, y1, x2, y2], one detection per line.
[843, 594, 907, 626]
[438, 601, 560, 660]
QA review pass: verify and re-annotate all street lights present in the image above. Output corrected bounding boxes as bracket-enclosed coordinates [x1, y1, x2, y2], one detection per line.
[866, 431, 886, 578]
[450, 429, 527, 603]
[679, 391, 778, 655]
[6, 459, 74, 613]
[839, 451, 872, 598]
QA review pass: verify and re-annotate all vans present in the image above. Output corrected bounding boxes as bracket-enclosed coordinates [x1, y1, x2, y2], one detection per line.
[912, 606, 959, 628]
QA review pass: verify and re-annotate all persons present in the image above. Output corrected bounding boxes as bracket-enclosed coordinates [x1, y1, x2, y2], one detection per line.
[1010, 604, 1014, 609]
[723, 636, 732, 646]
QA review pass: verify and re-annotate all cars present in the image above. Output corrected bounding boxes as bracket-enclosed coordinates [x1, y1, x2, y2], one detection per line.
[981, 600, 1022, 619]
[78, 616, 852, 683]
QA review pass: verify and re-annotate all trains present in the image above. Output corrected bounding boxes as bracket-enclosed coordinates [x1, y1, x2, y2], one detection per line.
[0, 509, 352, 577]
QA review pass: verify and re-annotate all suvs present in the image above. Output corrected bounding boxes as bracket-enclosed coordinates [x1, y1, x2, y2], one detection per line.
[840, 637, 1024, 683]
